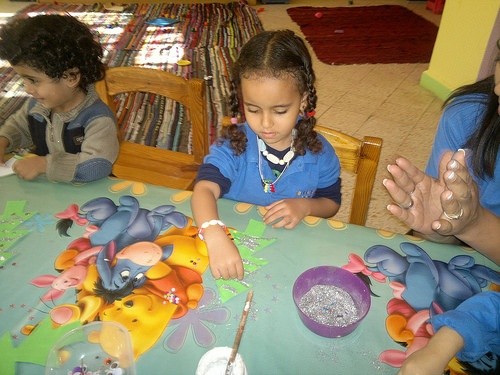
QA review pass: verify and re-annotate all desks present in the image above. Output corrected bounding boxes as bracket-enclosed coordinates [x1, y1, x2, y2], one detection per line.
[0, 152, 500, 375]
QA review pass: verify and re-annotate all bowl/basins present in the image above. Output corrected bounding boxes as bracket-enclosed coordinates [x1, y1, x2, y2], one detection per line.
[291, 265, 372, 338]
[46, 321, 137, 374]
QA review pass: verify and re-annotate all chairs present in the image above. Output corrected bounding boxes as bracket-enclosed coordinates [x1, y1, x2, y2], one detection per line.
[96, 67, 207, 192]
[222, 115, 383, 226]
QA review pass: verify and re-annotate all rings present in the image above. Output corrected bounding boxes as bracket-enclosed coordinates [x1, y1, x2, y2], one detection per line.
[405, 199, 414, 210]
[444, 209, 464, 220]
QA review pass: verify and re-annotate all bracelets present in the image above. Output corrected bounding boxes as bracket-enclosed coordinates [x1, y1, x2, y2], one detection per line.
[197, 220, 227, 241]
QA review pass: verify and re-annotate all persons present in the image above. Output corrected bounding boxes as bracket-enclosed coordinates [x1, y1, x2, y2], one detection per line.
[382, 36, 500, 272]
[0, 14, 119, 183]
[395, 289, 500, 375]
[190, 29, 342, 279]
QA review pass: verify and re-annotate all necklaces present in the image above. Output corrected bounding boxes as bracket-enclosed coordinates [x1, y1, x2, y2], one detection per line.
[258, 133, 293, 193]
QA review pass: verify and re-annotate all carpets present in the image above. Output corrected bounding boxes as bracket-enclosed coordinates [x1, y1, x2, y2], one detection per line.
[286, 5, 439, 65]
[0, 0, 265, 174]
